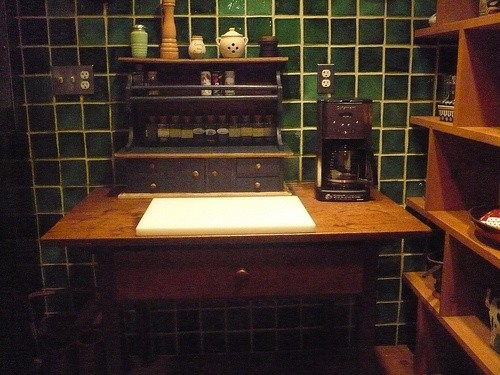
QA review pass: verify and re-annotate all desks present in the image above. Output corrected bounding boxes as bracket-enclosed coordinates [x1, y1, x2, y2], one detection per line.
[41, 180, 433, 375]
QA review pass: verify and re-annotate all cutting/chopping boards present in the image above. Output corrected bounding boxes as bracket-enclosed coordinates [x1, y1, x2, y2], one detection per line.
[136, 196, 315, 234]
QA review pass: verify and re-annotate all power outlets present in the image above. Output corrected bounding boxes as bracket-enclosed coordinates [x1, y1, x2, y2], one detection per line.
[317, 64, 335, 94]
[53, 65, 95, 96]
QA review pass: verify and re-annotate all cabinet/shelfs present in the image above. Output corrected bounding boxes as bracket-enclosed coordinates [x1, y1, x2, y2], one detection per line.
[111, 57, 293, 200]
[400, 0, 500, 375]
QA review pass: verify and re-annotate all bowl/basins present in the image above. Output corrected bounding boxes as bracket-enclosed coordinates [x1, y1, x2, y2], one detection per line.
[468, 205, 500, 246]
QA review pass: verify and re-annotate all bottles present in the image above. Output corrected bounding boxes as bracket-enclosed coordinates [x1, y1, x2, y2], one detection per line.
[241, 115, 253, 146]
[131, 25, 148, 58]
[181, 116, 193, 146]
[253, 115, 265, 146]
[158, 116, 171, 147]
[265, 115, 276, 146]
[206, 116, 216, 146]
[193, 116, 205, 146]
[170, 116, 181, 146]
[229, 116, 241, 145]
[216, 115, 229, 146]
[188, 36, 206, 59]
[145, 115, 158, 146]
